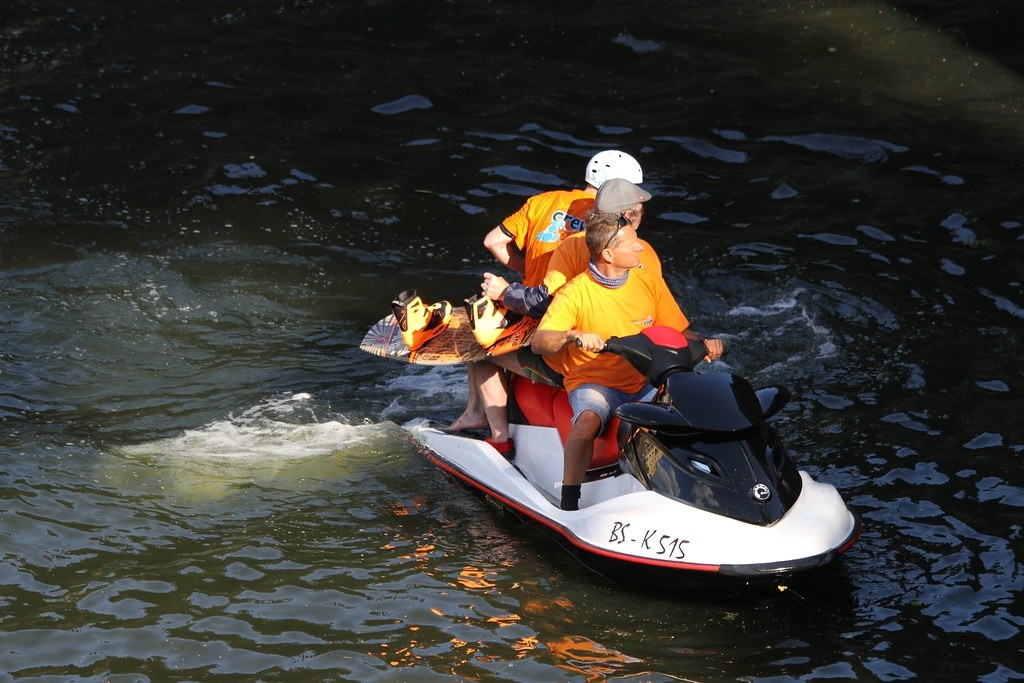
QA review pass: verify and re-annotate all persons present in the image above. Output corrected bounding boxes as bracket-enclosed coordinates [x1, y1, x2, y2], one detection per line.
[442, 149, 644, 433]
[531, 213, 723, 513]
[474, 178, 663, 460]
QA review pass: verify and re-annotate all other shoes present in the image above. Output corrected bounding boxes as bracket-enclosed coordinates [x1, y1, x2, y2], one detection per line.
[483, 435, 516, 461]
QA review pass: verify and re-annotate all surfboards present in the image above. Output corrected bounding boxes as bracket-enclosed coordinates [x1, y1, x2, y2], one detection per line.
[360, 305, 542, 366]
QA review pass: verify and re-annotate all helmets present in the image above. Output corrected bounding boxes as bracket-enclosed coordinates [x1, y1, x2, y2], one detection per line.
[585, 150, 644, 189]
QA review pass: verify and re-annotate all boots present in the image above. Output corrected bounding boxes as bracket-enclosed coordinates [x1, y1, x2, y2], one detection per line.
[464, 292, 529, 349]
[392, 289, 452, 350]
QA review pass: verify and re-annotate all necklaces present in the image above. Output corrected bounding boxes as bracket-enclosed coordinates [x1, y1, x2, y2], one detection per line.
[589, 257, 628, 289]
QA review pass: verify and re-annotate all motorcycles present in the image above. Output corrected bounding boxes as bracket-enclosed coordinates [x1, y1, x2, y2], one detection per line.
[401, 325, 861, 602]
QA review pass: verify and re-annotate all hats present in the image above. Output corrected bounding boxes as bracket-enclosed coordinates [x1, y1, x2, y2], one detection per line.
[594, 178, 652, 212]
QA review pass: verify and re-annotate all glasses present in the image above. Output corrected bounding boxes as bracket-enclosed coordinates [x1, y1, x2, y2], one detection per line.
[603, 216, 633, 254]
